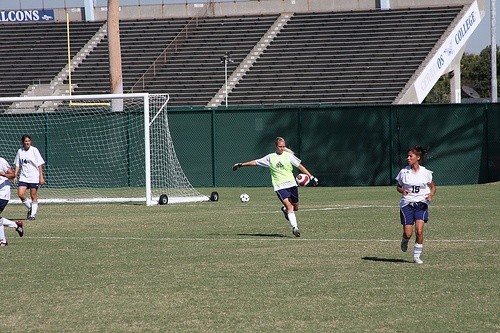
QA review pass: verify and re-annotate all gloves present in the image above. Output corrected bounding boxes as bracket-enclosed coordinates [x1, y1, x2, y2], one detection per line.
[310, 176, 318, 186]
[232, 163, 243, 171]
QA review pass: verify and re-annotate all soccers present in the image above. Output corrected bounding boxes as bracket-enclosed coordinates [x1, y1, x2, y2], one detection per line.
[239, 193, 250, 203]
[295, 174, 310, 186]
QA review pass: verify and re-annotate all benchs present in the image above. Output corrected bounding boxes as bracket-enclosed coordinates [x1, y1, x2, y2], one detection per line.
[0, 4, 463, 114]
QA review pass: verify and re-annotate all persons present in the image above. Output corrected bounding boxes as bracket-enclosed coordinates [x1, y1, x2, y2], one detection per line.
[395, 147, 436, 264]
[13, 134, 46, 220]
[0, 157, 23, 247]
[232, 137, 318, 237]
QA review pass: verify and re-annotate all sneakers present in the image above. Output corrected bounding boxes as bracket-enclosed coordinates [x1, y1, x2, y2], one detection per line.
[401, 236, 407, 252]
[0, 241, 7, 246]
[293, 228, 300, 236]
[282, 207, 288, 220]
[413, 257, 422, 264]
[26, 218, 35, 221]
[27, 208, 32, 219]
[15, 221, 23, 237]
